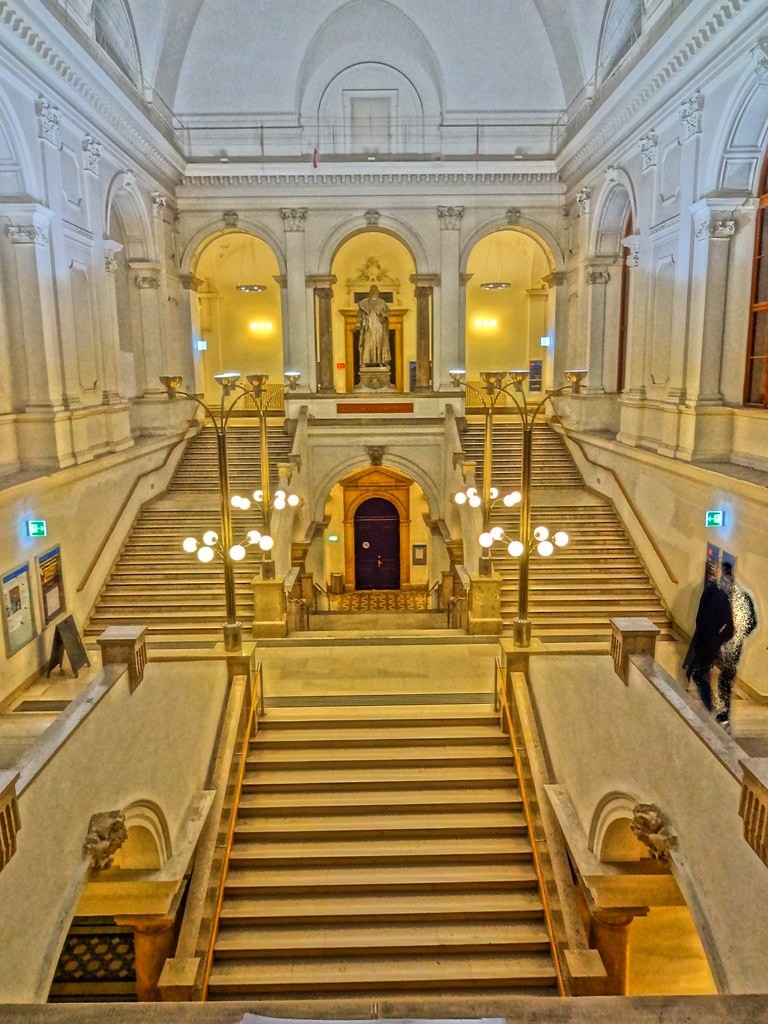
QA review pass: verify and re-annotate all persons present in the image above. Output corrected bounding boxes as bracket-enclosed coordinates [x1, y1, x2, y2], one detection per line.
[357, 285, 391, 367]
[680, 558, 757, 735]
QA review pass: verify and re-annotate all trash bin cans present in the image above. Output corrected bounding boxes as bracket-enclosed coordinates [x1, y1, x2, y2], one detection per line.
[331, 572, 344, 594]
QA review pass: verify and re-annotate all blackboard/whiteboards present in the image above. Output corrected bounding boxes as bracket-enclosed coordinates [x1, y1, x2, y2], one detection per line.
[56, 614, 87, 671]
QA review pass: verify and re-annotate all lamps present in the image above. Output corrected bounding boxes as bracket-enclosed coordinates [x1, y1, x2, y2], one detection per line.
[159, 373, 290, 652]
[449, 370, 522, 577]
[236, 235, 267, 292]
[217, 372, 303, 580]
[540, 336, 550, 347]
[480, 370, 587, 648]
[197, 340, 208, 351]
[480, 232, 511, 290]
